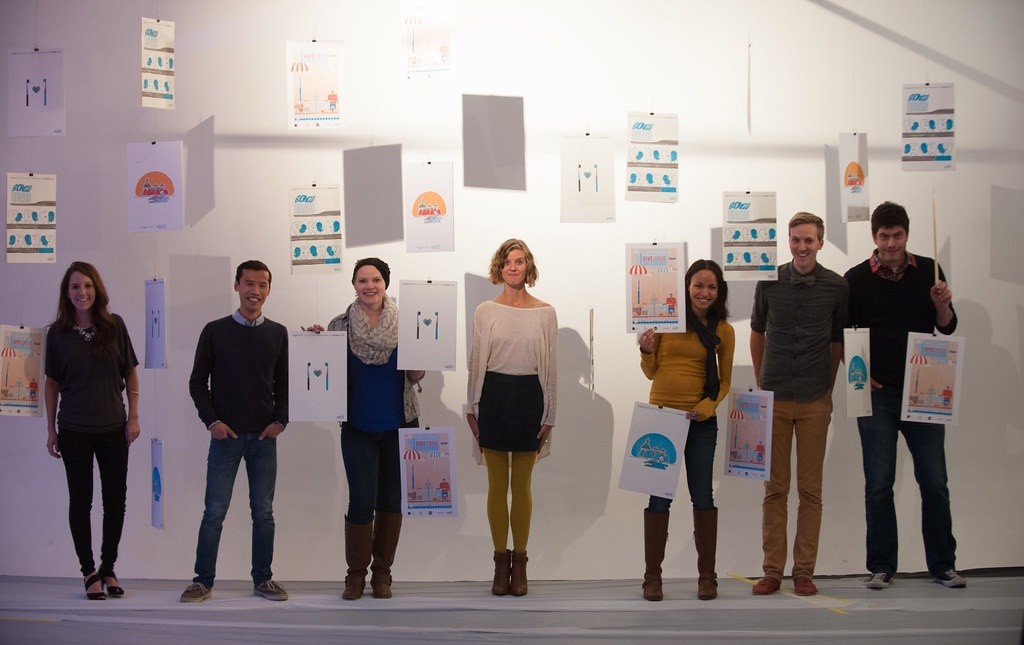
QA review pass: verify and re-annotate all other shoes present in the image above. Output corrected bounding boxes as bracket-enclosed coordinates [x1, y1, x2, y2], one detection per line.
[753, 575, 780, 594]
[793, 576, 817, 596]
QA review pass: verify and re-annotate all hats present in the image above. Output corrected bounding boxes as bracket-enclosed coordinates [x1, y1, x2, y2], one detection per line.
[354, 257, 390, 288]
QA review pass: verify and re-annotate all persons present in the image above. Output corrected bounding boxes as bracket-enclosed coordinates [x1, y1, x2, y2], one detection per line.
[639, 259, 735, 600]
[180, 261, 289, 602]
[466, 239, 558, 598]
[751, 212, 850, 596]
[42, 262, 142, 601]
[301, 257, 425, 600]
[843, 203, 965, 589]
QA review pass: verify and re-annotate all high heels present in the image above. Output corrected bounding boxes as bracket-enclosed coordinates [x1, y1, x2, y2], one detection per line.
[98, 567, 125, 595]
[83, 571, 106, 600]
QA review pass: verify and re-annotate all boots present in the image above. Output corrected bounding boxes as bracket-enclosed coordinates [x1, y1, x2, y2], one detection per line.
[693, 506, 718, 600]
[342, 514, 373, 599]
[492, 549, 511, 594]
[510, 549, 528, 596]
[642, 508, 670, 601]
[370, 509, 403, 598]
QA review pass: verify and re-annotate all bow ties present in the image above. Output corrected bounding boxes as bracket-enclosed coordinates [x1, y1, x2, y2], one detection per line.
[791, 274, 816, 287]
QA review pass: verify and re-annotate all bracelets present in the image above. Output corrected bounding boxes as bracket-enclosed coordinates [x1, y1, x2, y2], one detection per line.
[126, 391, 140, 396]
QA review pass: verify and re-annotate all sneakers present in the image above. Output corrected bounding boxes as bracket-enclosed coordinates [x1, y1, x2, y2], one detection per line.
[254, 581, 288, 600]
[934, 568, 968, 589]
[867, 571, 893, 588]
[180, 582, 211, 602]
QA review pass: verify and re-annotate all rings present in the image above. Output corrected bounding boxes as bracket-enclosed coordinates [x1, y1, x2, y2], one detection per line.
[643, 335, 647, 342]
